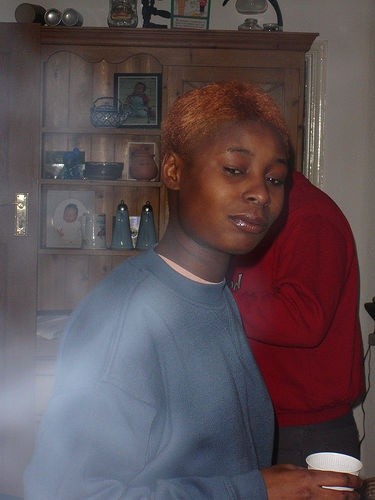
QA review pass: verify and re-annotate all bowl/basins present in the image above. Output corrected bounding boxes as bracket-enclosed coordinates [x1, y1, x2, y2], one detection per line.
[84, 161, 124, 179]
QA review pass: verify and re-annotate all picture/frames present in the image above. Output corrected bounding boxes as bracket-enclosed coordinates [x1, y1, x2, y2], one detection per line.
[112, 73, 162, 128]
[44, 192, 97, 249]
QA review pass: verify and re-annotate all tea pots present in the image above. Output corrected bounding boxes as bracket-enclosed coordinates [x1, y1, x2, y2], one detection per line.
[90, 97, 130, 128]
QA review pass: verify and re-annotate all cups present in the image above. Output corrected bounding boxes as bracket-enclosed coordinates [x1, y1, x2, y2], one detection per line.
[306, 452, 362, 491]
[64, 152, 78, 178]
[80, 213, 108, 249]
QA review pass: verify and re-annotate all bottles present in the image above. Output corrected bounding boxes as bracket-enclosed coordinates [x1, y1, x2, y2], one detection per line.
[135, 200, 157, 249]
[111, 199, 133, 248]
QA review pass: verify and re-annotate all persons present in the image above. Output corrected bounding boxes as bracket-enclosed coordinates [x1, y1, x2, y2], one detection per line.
[16, 80, 368, 500]
[222, 169, 365, 469]
[53, 204, 85, 249]
[122, 82, 152, 117]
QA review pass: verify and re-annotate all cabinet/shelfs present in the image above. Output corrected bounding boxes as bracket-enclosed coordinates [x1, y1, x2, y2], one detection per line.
[1, 21, 320, 500]
